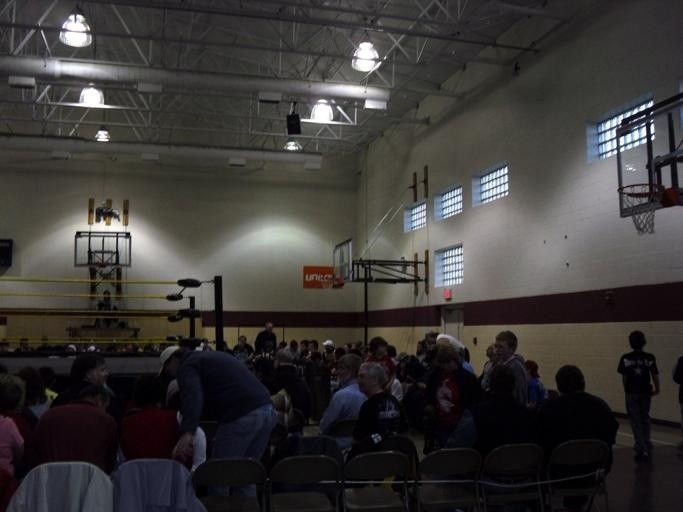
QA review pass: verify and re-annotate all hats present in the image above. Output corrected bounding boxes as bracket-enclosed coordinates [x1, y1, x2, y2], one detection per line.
[322, 339, 334, 346]
[157, 345, 180, 374]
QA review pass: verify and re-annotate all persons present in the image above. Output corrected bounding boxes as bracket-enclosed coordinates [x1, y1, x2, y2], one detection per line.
[230, 336, 254, 361]
[1, 375, 26, 508]
[165, 379, 206, 476]
[343, 363, 420, 504]
[1, 338, 15, 353]
[524, 360, 545, 405]
[202, 338, 215, 351]
[413, 332, 471, 365]
[94, 290, 120, 328]
[400, 332, 473, 455]
[48, 353, 125, 424]
[255, 321, 277, 356]
[15, 338, 35, 352]
[367, 337, 396, 390]
[190, 338, 205, 351]
[446, 365, 537, 497]
[222, 341, 233, 355]
[323, 347, 347, 408]
[537, 364, 620, 507]
[158, 346, 274, 497]
[260, 350, 314, 456]
[478, 346, 500, 381]
[319, 354, 368, 435]
[36, 336, 54, 351]
[673, 354, 683, 410]
[23, 372, 119, 477]
[386, 345, 399, 366]
[54, 337, 177, 352]
[120, 374, 179, 464]
[277, 341, 370, 369]
[617, 331, 660, 453]
[158, 338, 196, 411]
[481, 331, 528, 407]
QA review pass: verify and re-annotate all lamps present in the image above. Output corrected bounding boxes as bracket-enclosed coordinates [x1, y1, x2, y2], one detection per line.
[58, 9, 92, 48]
[309, 97, 334, 123]
[94, 126, 111, 143]
[79, 80, 105, 110]
[351, 40, 379, 72]
[282, 137, 303, 153]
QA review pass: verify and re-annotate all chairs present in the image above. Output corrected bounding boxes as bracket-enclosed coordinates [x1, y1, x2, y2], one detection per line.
[198, 421, 218, 441]
[323, 417, 359, 438]
[110, 459, 196, 512]
[5, 462, 113, 512]
[263, 435, 345, 473]
[539, 390, 559, 406]
[267, 454, 340, 512]
[544, 438, 609, 512]
[341, 450, 412, 512]
[413, 448, 479, 512]
[347, 436, 419, 476]
[193, 457, 269, 512]
[479, 444, 544, 512]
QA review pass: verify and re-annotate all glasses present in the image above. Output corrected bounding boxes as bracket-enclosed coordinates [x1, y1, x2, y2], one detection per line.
[356, 372, 365, 379]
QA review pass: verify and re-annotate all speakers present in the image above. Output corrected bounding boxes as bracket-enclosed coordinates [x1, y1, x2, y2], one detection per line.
[287, 114, 301, 135]
[1, 239, 13, 267]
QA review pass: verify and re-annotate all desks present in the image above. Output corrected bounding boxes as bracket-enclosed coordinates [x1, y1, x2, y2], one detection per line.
[68, 327, 140, 339]
[0, 351, 163, 379]
